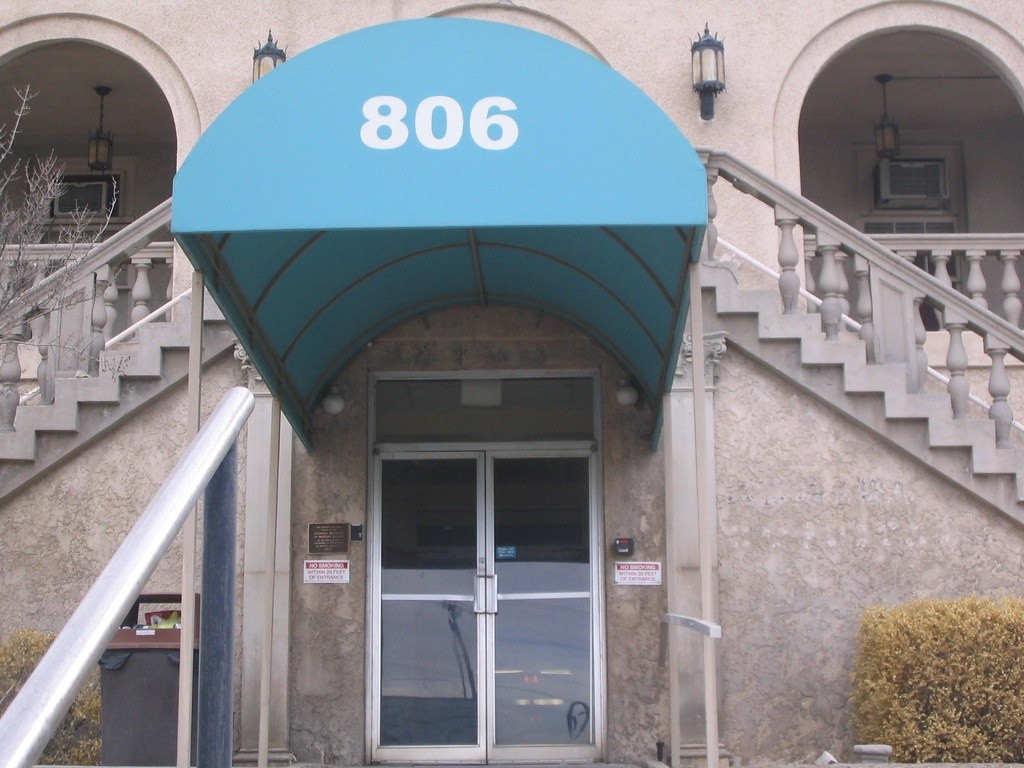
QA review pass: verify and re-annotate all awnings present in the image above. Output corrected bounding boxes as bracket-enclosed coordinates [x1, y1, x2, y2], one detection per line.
[171, 17, 708, 455]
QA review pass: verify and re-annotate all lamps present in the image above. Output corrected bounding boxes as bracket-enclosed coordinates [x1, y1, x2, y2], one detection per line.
[322, 378, 345, 416]
[615, 378, 646, 413]
[687, 21, 727, 121]
[88, 86, 115, 178]
[253, 28, 289, 83]
[874, 74, 901, 160]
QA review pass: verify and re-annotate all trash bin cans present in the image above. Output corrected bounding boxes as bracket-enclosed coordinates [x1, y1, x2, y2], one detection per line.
[95, 591, 201, 768]
